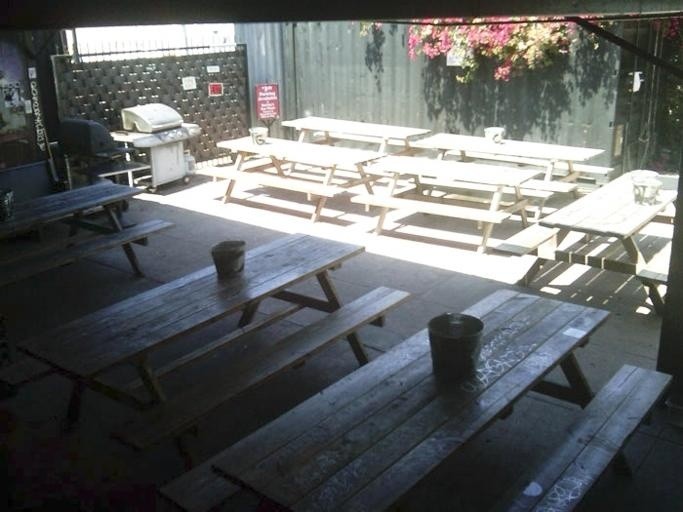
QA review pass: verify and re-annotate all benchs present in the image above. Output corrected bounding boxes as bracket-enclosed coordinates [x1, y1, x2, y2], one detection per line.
[505, 364, 672, 511]
[187, 119, 606, 255]
[488, 222, 561, 291]
[0, 215, 178, 296]
[145, 281, 415, 462]
[637, 237, 674, 320]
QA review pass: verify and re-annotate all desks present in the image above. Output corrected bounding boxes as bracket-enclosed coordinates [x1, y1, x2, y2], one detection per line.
[538, 166, 679, 239]
[13, 229, 369, 433]
[0, 179, 146, 280]
[156, 287, 613, 511]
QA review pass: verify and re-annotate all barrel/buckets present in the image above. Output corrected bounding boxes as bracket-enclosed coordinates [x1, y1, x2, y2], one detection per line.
[630, 175, 662, 203]
[485, 126, 504, 142]
[428, 311, 485, 377]
[211, 240, 247, 279]
[248, 127, 268, 144]
